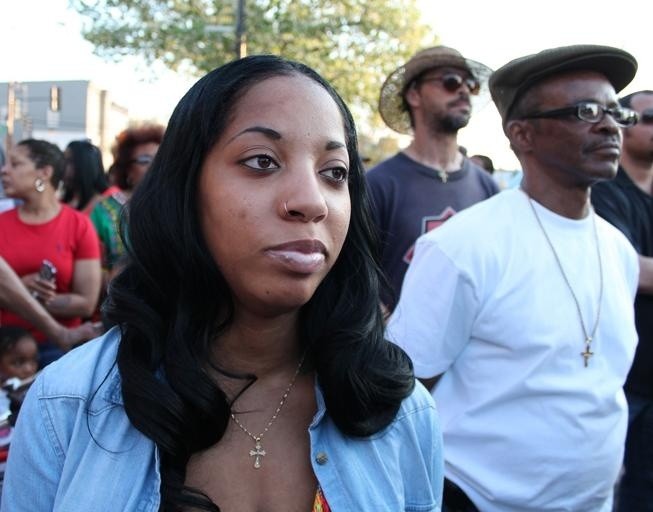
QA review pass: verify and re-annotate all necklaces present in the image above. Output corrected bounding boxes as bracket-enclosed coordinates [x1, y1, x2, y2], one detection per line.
[436, 167, 450, 184]
[225, 359, 300, 474]
[523, 192, 606, 371]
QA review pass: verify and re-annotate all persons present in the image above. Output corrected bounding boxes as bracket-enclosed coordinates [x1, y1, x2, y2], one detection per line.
[0, 53, 447, 512]
[360, 42, 508, 392]
[589, 89, 653, 510]
[0, 120, 168, 487]
[376, 45, 641, 510]
[470, 154, 495, 177]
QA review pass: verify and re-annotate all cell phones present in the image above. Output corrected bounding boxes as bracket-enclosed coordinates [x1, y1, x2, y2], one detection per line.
[40, 259, 57, 280]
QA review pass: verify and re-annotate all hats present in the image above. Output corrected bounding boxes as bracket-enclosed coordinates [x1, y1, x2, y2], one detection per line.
[378, 46, 495, 136]
[488, 45, 638, 138]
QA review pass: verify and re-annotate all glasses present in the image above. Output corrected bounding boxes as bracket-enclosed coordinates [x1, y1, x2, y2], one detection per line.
[418, 73, 481, 95]
[129, 154, 154, 165]
[519, 101, 653, 129]
